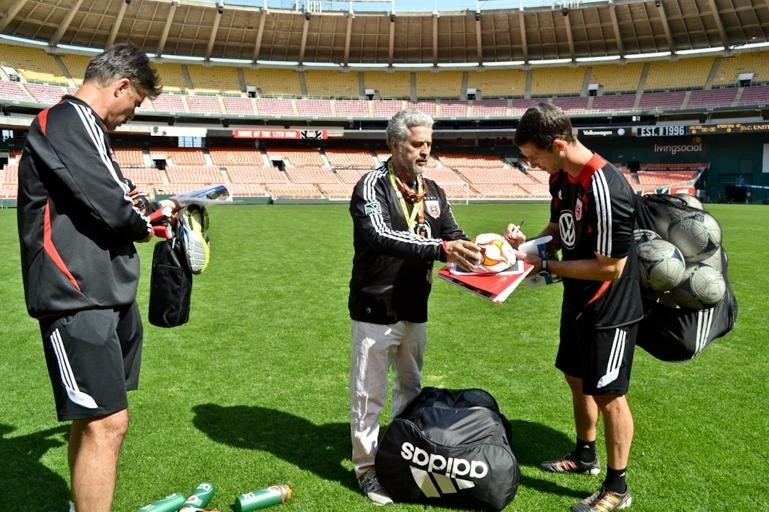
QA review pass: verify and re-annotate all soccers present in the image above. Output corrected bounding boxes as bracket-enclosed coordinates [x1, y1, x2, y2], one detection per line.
[637, 239, 686, 294]
[463, 232, 517, 274]
[668, 192, 704, 211]
[632, 227, 660, 245]
[700, 246, 730, 275]
[672, 262, 728, 310]
[666, 208, 723, 264]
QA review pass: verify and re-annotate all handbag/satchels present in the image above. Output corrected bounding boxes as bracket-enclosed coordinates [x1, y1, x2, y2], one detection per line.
[375, 386, 519, 511]
[149, 241, 192, 327]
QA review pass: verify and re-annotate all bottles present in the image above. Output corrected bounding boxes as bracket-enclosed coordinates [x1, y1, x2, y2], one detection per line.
[186, 480, 216, 509]
[233, 483, 294, 510]
[134, 491, 185, 512]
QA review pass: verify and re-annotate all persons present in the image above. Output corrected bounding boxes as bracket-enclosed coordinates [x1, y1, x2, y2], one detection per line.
[17, 41, 155, 512]
[505, 102, 645, 512]
[348, 107, 482, 507]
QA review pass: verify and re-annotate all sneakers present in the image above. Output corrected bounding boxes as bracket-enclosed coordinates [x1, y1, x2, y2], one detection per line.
[358, 472, 394, 508]
[540, 451, 601, 475]
[569, 482, 632, 512]
[178, 208, 211, 274]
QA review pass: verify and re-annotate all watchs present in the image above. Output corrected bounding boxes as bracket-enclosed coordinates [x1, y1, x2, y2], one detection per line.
[538, 258, 549, 276]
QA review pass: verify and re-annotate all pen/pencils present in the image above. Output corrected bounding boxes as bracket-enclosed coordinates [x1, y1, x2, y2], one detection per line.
[507, 219, 527, 245]
